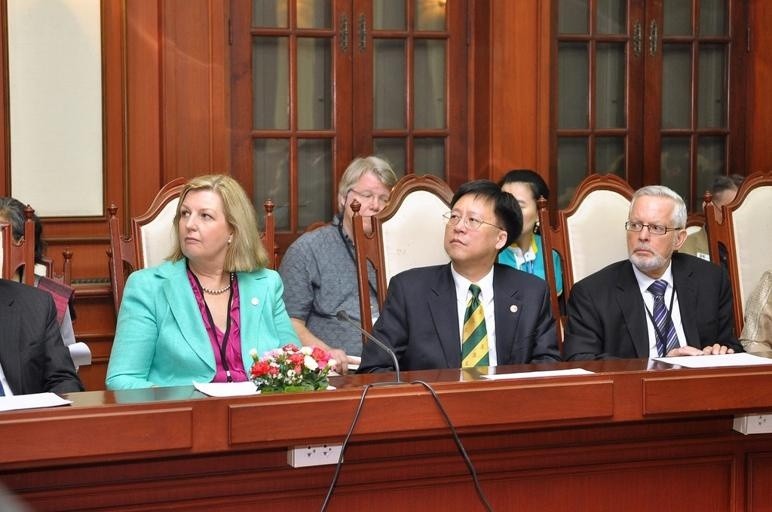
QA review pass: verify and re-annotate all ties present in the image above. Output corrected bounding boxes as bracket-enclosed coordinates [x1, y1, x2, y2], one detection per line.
[646, 277, 682, 358]
[460, 283, 491, 370]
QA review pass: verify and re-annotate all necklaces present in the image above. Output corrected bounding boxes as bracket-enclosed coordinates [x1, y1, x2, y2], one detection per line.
[190, 269, 234, 295]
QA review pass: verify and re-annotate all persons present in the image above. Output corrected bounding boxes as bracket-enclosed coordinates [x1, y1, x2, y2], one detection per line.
[104, 175, 303, 392]
[738, 269, 772, 360]
[0, 279, 83, 397]
[493, 168, 563, 299]
[353, 179, 561, 375]
[0, 196, 78, 374]
[677, 174, 744, 277]
[560, 184, 744, 362]
[279, 155, 399, 377]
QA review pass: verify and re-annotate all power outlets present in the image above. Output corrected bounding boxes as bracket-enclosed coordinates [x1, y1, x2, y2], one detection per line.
[286, 443, 344, 470]
[732, 414, 772, 436]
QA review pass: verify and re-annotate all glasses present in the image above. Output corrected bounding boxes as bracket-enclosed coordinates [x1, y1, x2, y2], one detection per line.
[624, 218, 684, 236]
[349, 186, 389, 207]
[441, 209, 505, 234]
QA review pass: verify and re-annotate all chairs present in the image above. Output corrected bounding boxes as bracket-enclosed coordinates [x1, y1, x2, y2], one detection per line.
[350, 173, 498, 346]
[261, 221, 332, 272]
[106, 234, 129, 289]
[34, 247, 74, 288]
[535, 173, 636, 356]
[106, 174, 275, 325]
[673, 213, 711, 263]
[0, 204, 36, 287]
[702, 167, 772, 342]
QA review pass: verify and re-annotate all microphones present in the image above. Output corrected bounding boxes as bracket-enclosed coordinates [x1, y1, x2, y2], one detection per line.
[336, 310, 408, 385]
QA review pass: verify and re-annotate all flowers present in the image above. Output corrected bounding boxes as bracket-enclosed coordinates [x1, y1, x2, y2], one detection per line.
[249, 342, 343, 395]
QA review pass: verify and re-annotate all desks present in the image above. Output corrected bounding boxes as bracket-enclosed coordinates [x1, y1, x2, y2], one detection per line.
[0, 350, 772, 512]
